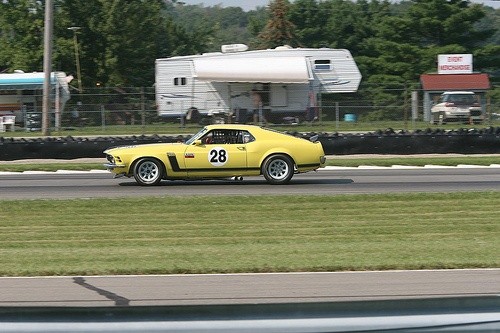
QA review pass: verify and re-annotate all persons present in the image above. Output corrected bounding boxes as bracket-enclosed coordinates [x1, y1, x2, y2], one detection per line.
[251, 91, 267, 123]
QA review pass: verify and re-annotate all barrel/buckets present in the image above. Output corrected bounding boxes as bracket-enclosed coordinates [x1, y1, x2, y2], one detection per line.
[344, 113, 357, 123]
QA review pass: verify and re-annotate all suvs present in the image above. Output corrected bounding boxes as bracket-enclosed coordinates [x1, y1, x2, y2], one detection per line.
[430, 91, 479, 121]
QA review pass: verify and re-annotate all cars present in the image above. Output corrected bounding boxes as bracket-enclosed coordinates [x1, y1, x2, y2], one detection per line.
[102, 124, 325, 185]
